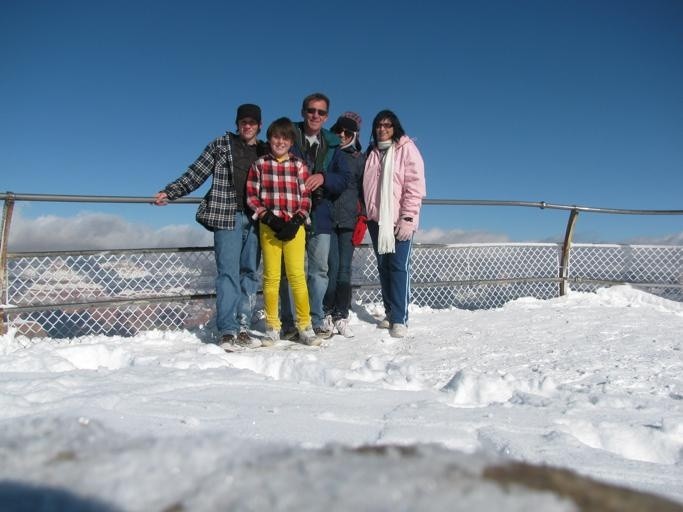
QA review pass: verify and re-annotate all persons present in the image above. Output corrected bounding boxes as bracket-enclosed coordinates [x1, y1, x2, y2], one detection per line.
[244, 115, 328, 347]
[362, 107, 429, 338]
[266, 93, 351, 340]
[148, 104, 272, 352]
[330, 108, 366, 339]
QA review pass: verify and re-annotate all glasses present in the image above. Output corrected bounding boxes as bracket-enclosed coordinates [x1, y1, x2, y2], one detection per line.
[335, 127, 353, 137]
[305, 107, 326, 116]
[376, 122, 393, 128]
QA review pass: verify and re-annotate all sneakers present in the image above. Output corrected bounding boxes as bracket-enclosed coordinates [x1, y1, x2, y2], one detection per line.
[261, 330, 280, 347]
[284, 315, 354, 346]
[379, 317, 391, 328]
[219, 334, 237, 351]
[391, 323, 407, 337]
[237, 332, 261, 349]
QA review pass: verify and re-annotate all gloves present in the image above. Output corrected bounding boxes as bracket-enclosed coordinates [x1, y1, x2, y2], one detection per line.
[262, 210, 305, 240]
[351, 215, 367, 245]
[394, 217, 415, 242]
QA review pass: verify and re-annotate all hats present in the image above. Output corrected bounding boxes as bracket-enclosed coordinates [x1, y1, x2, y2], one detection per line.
[237, 104, 261, 122]
[337, 111, 361, 129]
